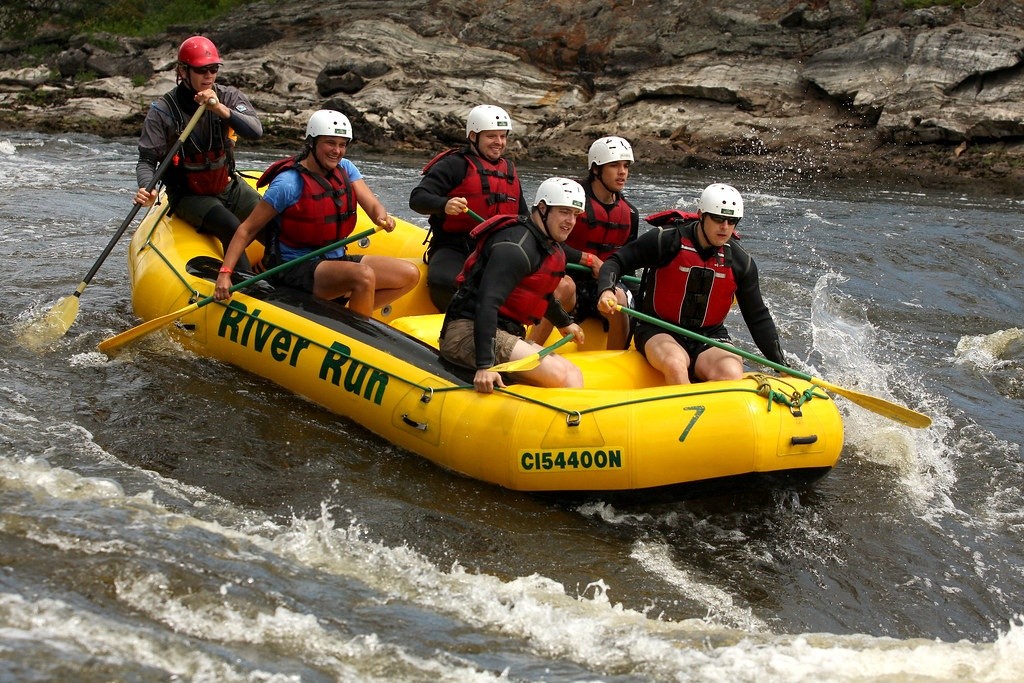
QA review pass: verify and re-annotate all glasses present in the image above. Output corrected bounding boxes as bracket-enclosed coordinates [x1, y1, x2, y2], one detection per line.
[181, 62, 221, 75]
[708, 213, 739, 225]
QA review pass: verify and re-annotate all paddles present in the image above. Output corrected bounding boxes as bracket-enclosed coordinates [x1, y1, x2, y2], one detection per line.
[463, 207, 486, 223]
[52, 97, 218, 334]
[485, 331, 577, 374]
[566, 262, 642, 284]
[97, 220, 389, 355]
[608, 299, 933, 429]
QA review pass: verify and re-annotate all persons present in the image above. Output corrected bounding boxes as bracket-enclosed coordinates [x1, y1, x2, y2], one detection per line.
[132, 36, 266, 270]
[214, 110, 420, 318]
[597, 183, 792, 386]
[527, 137, 640, 350]
[408, 104, 531, 316]
[438, 177, 584, 393]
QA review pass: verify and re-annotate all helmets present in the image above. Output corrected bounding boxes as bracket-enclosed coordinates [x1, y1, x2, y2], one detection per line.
[465, 104, 513, 140]
[698, 183, 744, 219]
[587, 136, 635, 171]
[533, 176, 586, 215]
[177, 36, 224, 67]
[306, 108, 353, 145]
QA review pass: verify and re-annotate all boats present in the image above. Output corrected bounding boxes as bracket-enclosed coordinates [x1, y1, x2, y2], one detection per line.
[127, 168, 844, 503]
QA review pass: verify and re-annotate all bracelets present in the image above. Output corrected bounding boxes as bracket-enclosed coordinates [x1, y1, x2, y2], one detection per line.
[219, 266, 233, 273]
[585, 253, 593, 266]
